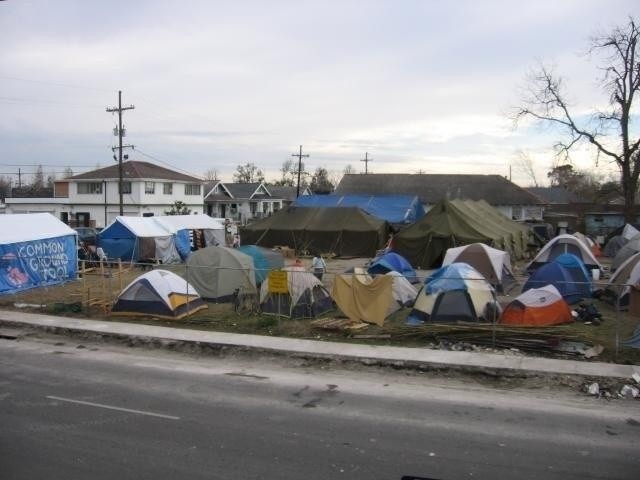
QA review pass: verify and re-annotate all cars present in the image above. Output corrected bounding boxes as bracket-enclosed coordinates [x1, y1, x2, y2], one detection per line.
[74, 228, 98, 247]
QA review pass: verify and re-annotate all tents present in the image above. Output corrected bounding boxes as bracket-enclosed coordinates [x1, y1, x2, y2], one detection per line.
[1, 193, 640, 327]
